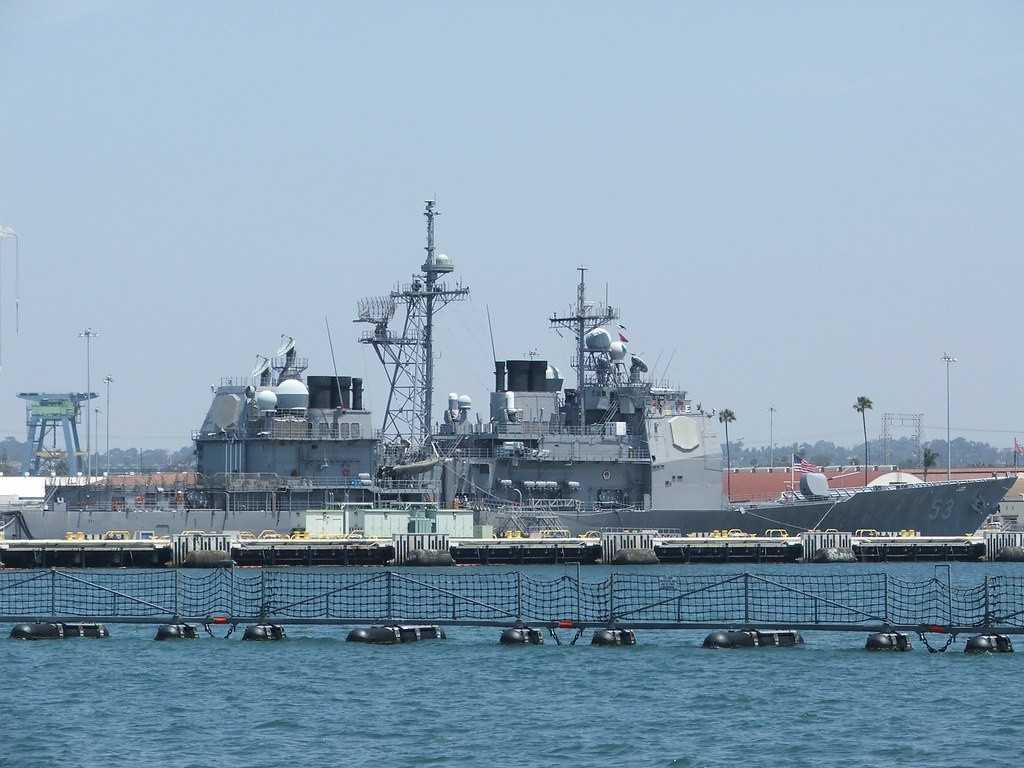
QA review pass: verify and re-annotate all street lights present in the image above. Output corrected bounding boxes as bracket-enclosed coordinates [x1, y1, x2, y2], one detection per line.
[78, 328, 100, 485]
[103, 374, 115, 484]
[765, 406, 778, 467]
[91, 406, 102, 483]
[940, 352, 957, 481]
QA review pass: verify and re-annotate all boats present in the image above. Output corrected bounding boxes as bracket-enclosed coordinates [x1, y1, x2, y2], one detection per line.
[0, 194, 1021, 570]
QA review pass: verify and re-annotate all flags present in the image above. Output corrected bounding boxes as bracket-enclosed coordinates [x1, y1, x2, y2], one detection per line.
[618, 323, 629, 342]
[1014, 439, 1023, 456]
[791, 455, 817, 473]
